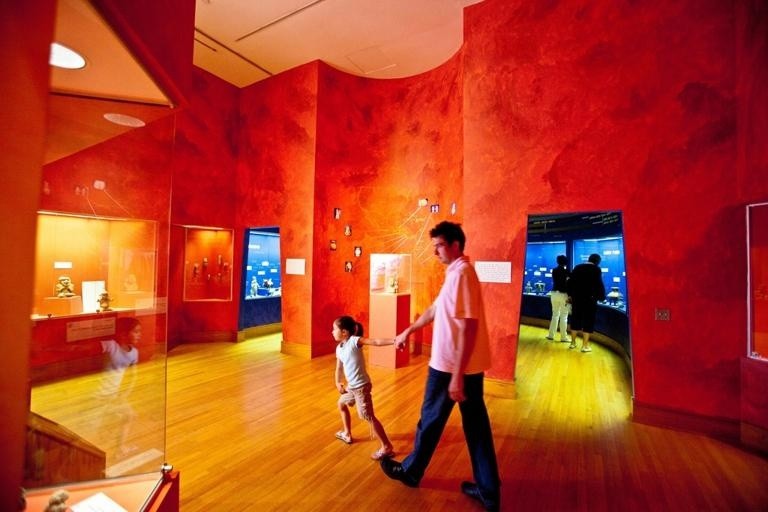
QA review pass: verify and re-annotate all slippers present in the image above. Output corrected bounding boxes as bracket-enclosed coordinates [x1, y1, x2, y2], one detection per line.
[372, 451, 395, 460]
[336, 432, 352, 443]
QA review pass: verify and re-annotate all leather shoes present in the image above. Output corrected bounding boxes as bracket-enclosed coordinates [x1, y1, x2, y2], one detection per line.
[380, 458, 420, 487]
[461, 481, 481, 499]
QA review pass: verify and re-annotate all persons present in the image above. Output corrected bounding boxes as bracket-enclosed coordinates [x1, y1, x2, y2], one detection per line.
[566, 253, 606, 352]
[379, 220, 502, 512]
[331, 315, 406, 461]
[544, 255, 572, 343]
[67, 315, 141, 462]
